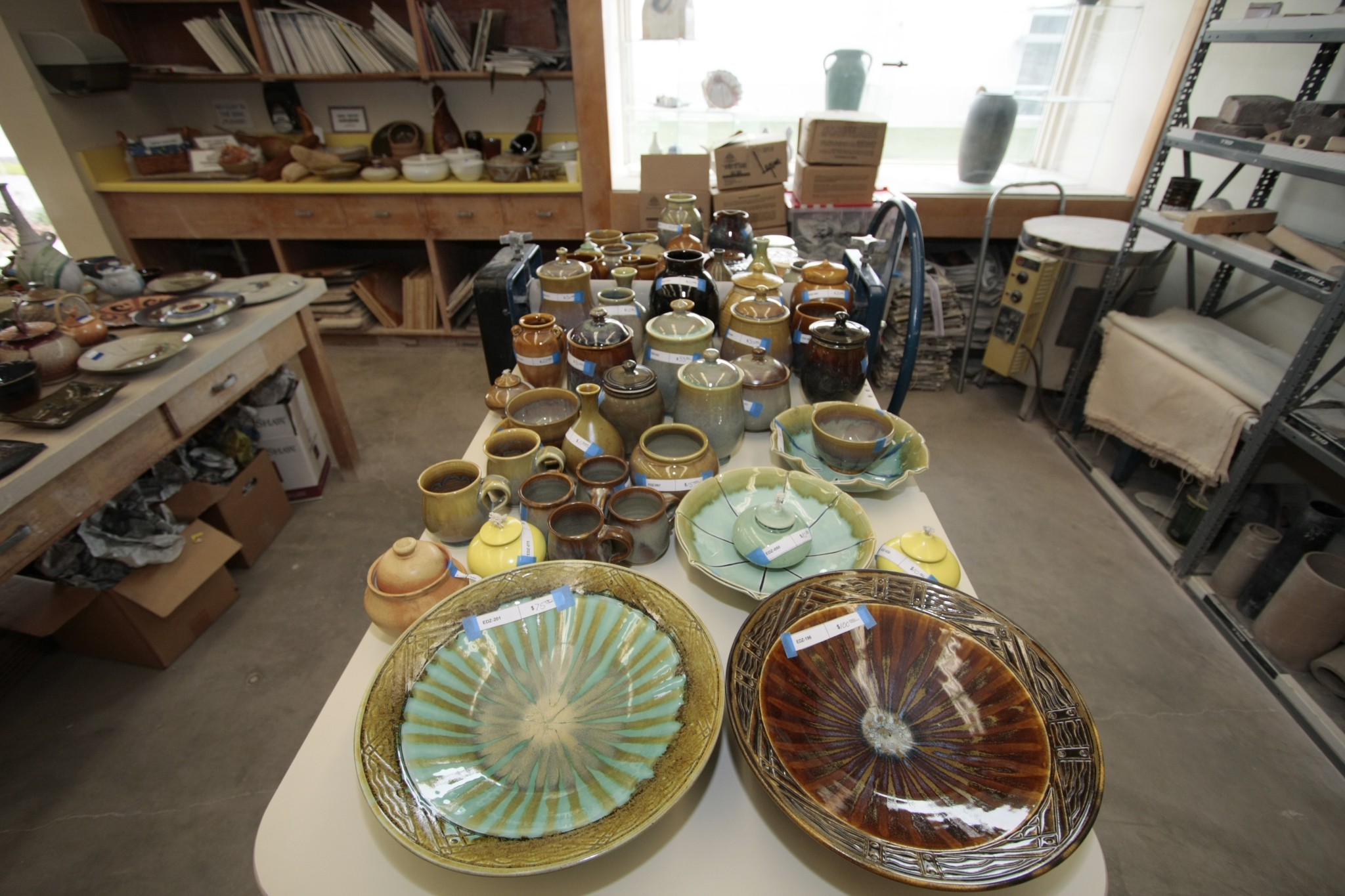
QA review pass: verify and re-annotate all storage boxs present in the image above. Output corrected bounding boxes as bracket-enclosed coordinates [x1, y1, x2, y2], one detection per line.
[0, 377, 333, 671]
[639, 113, 887, 258]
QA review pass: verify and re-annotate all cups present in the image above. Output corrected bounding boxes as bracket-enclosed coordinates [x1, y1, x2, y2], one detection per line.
[417, 460, 512, 544]
[604, 486, 681, 566]
[519, 472, 579, 537]
[547, 503, 634, 565]
[484, 429, 565, 505]
[574, 455, 631, 511]
[492, 386, 579, 447]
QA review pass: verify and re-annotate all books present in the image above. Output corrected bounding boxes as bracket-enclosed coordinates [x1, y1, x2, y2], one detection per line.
[183, 1, 572, 75]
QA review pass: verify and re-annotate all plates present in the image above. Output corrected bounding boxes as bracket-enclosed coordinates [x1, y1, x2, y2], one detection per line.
[354, 559, 1106, 890]
[675, 401, 930, 602]
[0, 270, 305, 483]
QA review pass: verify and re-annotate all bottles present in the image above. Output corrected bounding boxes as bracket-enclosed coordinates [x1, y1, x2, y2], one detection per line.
[485, 191, 931, 498]
[365, 515, 547, 641]
[431, 85, 547, 164]
[732, 497, 961, 590]
[823, 48, 872, 111]
[958, 90, 1017, 185]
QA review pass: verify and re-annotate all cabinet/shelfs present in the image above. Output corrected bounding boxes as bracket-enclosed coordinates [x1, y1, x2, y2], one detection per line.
[1044, 0, 1345, 769]
[0, 0, 617, 337]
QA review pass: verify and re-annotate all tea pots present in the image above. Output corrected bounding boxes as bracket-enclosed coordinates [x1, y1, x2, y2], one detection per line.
[0, 182, 144, 385]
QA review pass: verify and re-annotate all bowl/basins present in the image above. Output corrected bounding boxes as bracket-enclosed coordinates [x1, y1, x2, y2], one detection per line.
[1, 361, 42, 414]
[812, 403, 895, 475]
[310, 142, 581, 186]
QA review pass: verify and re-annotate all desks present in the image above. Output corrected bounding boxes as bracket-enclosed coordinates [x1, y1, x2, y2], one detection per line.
[0, 278, 358, 582]
[252, 275, 1105, 896]
